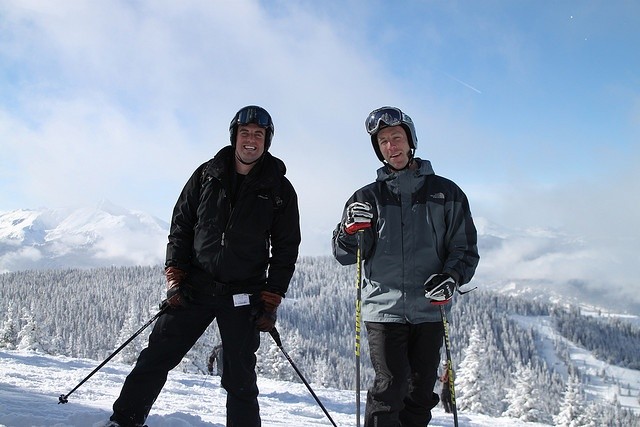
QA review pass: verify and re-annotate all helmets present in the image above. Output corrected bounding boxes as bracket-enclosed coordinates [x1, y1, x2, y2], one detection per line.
[229, 106, 274, 153]
[371, 107, 418, 161]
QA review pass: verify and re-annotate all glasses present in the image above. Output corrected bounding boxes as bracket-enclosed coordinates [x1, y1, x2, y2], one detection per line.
[229, 106, 274, 134]
[366, 107, 412, 134]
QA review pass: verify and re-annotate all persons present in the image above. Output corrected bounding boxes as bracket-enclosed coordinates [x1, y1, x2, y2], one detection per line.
[331, 106, 480, 426]
[106, 105, 301, 426]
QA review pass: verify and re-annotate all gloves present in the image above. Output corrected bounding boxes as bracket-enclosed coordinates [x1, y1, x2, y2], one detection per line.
[250, 291, 282, 332]
[166, 267, 194, 309]
[341, 202, 374, 235]
[424, 273, 458, 305]
